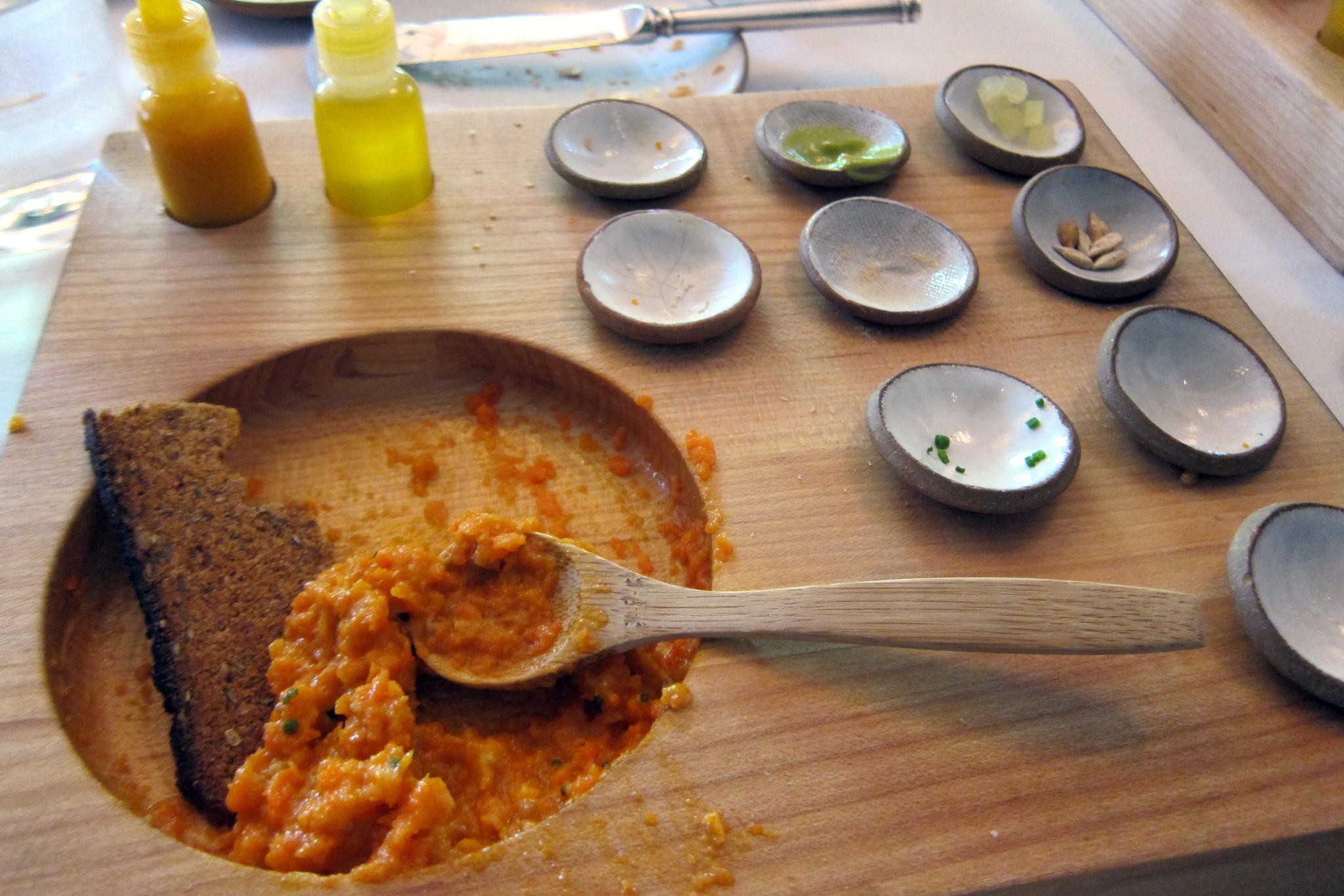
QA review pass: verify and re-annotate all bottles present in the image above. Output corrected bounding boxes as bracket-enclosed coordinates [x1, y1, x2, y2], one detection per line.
[122, 0, 275, 228]
[311, 0, 432, 218]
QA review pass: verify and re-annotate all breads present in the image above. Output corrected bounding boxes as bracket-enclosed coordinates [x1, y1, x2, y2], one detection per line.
[83, 401, 335, 824]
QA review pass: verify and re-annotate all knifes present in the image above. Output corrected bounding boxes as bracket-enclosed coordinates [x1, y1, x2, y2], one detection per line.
[397, 0, 921, 65]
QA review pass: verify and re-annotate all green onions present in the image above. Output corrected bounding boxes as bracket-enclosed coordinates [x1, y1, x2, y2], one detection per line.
[927, 398, 1046, 473]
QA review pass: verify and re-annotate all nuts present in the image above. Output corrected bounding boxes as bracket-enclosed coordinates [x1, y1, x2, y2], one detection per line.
[1053, 211, 1126, 269]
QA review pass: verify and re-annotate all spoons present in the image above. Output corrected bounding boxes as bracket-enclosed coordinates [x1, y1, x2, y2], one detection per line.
[409, 532, 1208, 688]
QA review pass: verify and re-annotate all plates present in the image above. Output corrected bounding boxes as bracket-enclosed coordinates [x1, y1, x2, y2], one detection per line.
[1097, 304, 1287, 476]
[307, 0, 748, 109]
[1227, 501, 1344, 707]
[934, 63, 1086, 176]
[1012, 164, 1180, 300]
[546, 99, 708, 198]
[207, 0, 322, 19]
[799, 196, 980, 326]
[576, 208, 763, 345]
[756, 100, 911, 186]
[866, 362, 1082, 514]
